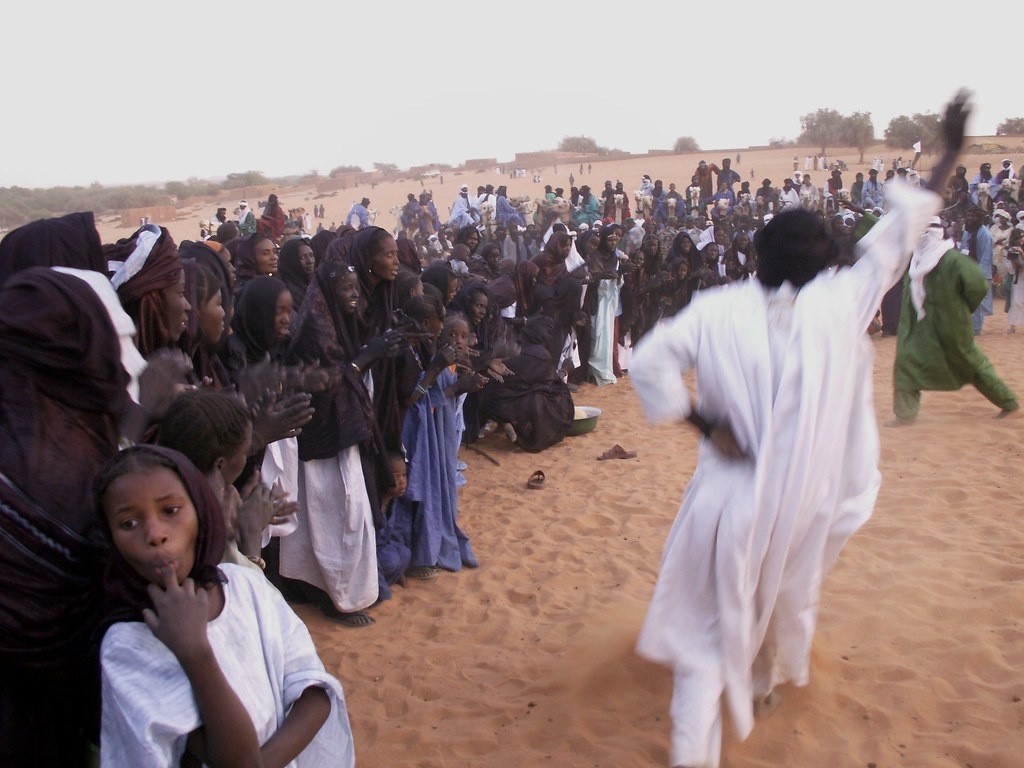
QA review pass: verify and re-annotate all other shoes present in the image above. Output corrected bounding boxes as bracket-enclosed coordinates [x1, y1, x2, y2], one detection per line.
[417, 567, 438, 579]
[324, 611, 375, 627]
[567, 382, 583, 392]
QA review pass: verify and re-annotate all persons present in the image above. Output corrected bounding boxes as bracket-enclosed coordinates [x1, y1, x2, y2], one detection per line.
[630, 85, 972, 768]
[1, 138, 1023, 630]
[99, 446, 358, 768]
[0, 268, 202, 768]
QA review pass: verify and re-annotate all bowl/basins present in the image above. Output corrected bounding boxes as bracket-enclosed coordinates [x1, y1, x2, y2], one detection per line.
[568, 405, 601, 436]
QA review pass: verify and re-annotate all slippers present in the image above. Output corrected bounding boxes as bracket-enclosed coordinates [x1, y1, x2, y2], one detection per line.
[596, 444, 637, 460]
[527, 470, 547, 490]
